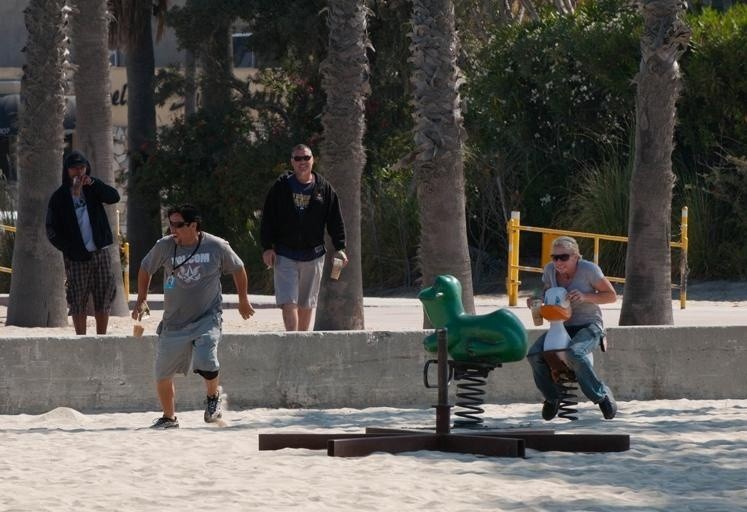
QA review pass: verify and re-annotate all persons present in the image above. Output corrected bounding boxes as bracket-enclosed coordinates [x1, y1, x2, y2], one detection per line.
[260, 144, 348, 331]
[527, 236, 618, 420]
[131, 204, 255, 429]
[45, 151, 120, 335]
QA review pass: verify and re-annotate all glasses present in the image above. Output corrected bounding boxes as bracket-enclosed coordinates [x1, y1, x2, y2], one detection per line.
[292, 156, 312, 161]
[551, 254, 569, 262]
[170, 222, 188, 228]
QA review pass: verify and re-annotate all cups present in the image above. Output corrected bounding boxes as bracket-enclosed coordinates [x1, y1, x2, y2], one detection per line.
[529, 298, 547, 327]
[133, 313, 147, 338]
[72, 175, 83, 196]
[330, 259, 344, 281]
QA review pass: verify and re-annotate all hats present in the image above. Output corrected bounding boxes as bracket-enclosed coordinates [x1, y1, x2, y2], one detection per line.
[67, 155, 87, 168]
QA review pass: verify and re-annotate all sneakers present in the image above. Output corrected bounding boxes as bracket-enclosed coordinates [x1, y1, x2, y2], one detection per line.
[149, 416, 179, 428]
[598, 385, 617, 419]
[542, 394, 559, 421]
[204, 385, 223, 422]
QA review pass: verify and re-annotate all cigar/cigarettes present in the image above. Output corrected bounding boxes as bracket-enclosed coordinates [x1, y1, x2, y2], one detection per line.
[267, 265, 271, 270]
